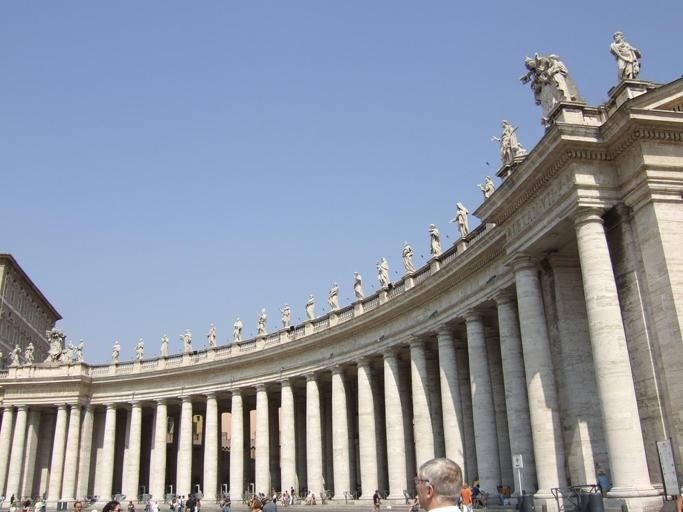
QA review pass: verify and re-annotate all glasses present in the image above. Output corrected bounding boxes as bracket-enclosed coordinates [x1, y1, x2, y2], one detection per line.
[413, 476, 430, 484]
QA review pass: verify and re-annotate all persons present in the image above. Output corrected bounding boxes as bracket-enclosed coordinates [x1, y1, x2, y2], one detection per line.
[412, 455, 463, 512]
[400, 241, 414, 271]
[0, 493, 46, 512]
[281, 304, 290, 327]
[306, 294, 315, 319]
[376, 257, 389, 287]
[447, 200, 470, 237]
[427, 223, 440, 254]
[246, 486, 316, 512]
[11, 343, 35, 365]
[170, 493, 201, 512]
[374, 490, 381, 512]
[160, 334, 168, 356]
[207, 323, 216, 346]
[328, 282, 339, 309]
[402, 489, 419, 512]
[532, 53, 573, 120]
[63, 339, 84, 363]
[258, 308, 266, 333]
[232, 318, 243, 340]
[73, 499, 160, 512]
[609, 27, 643, 81]
[489, 119, 519, 164]
[182, 328, 193, 352]
[112, 341, 121, 363]
[135, 338, 144, 359]
[352, 272, 364, 299]
[220, 494, 232, 511]
[475, 175, 494, 201]
[459, 483, 510, 512]
